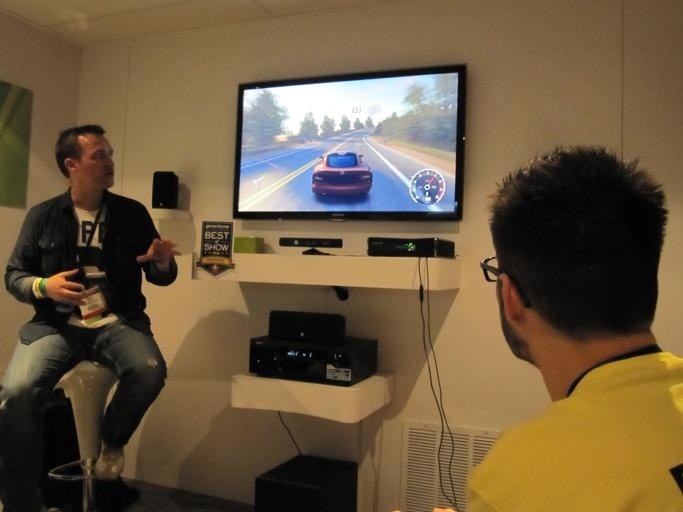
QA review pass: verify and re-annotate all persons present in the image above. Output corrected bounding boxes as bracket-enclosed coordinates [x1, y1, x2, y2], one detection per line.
[0, 124, 183, 512]
[463, 147, 682, 512]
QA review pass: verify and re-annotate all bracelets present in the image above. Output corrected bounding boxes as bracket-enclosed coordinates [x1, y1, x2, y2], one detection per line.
[38, 277, 47, 297]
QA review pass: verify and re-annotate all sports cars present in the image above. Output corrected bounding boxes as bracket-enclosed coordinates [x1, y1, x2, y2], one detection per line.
[312, 151, 373, 198]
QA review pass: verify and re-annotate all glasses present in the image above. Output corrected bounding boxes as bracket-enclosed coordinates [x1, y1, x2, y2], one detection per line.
[479, 256, 531, 308]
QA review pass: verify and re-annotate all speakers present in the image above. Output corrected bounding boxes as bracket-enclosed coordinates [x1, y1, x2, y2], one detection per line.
[150, 170, 178, 208]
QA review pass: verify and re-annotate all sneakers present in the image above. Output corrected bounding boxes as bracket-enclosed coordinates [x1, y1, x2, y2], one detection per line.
[94, 440, 125, 480]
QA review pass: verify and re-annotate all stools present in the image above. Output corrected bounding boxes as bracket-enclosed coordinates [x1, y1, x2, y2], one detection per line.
[47, 362, 118, 512]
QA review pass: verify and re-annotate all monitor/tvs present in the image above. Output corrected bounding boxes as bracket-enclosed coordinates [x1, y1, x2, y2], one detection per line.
[231, 63, 467, 223]
